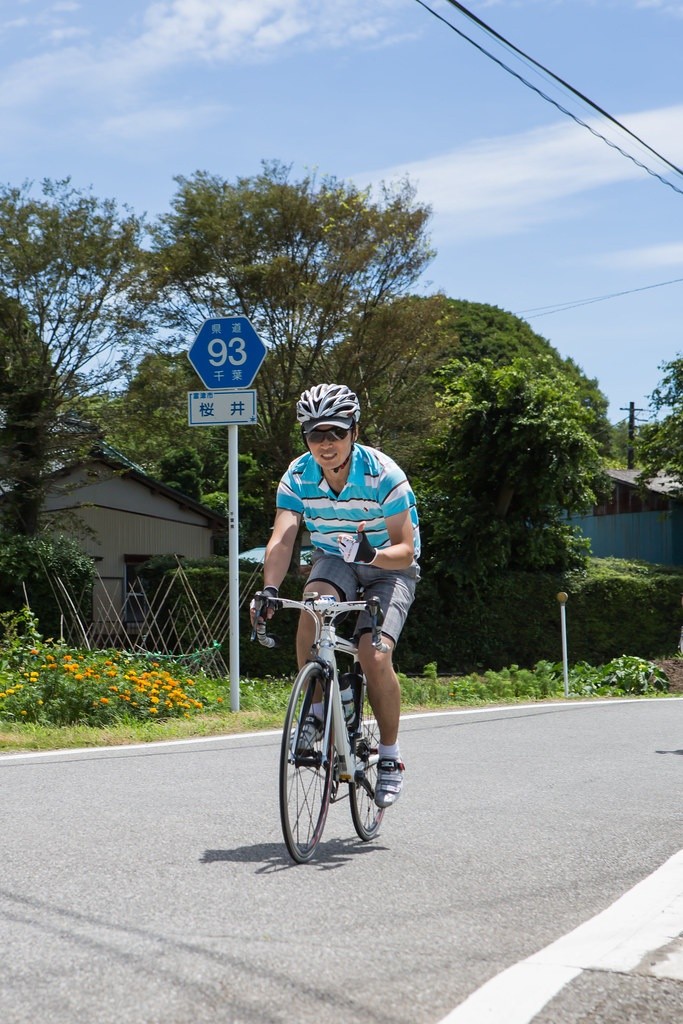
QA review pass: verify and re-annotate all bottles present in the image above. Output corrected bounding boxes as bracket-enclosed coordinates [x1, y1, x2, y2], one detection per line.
[337, 669, 356, 729]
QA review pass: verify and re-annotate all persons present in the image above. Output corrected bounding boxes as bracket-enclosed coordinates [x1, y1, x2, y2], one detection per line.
[250, 383, 422, 809]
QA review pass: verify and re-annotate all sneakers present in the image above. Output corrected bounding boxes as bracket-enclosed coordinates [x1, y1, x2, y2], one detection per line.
[374, 756, 405, 808]
[289, 714, 324, 753]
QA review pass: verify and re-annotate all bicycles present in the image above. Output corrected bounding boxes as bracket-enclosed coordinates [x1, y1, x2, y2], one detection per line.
[251, 588, 383, 867]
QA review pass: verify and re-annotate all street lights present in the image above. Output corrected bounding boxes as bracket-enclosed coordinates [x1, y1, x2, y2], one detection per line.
[557, 591, 571, 698]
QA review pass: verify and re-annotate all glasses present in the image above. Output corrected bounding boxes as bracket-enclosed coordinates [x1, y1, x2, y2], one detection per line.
[304, 428, 348, 443]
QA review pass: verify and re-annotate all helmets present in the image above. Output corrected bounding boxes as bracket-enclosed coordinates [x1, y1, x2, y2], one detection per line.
[297, 383, 361, 432]
